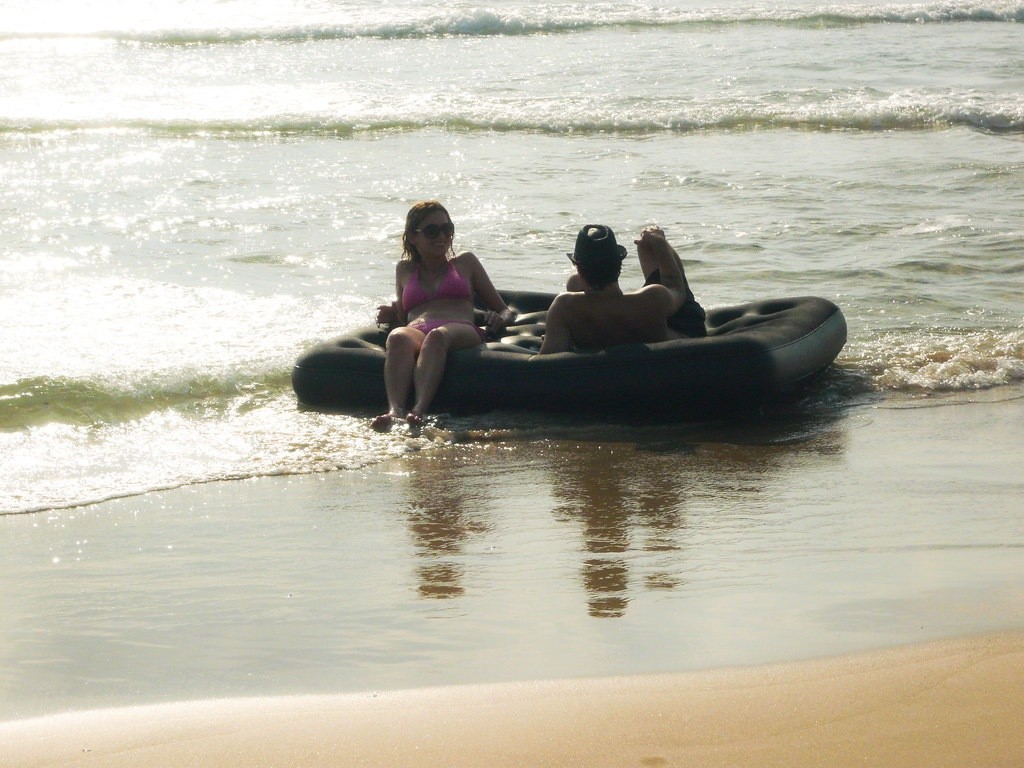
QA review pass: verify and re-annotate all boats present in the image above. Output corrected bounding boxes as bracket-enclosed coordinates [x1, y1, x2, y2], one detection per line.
[287, 284, 849, 423]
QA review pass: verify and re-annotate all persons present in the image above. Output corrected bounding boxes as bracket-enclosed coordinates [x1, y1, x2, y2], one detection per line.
[370, 200, 513, 432]
[538, 224, 707, 355]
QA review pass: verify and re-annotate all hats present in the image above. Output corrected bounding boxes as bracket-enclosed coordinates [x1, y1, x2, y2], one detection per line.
[567, 224, 628, 266]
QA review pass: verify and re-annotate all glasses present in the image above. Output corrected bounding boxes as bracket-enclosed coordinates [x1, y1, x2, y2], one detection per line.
[413, 222, 454, 240]
